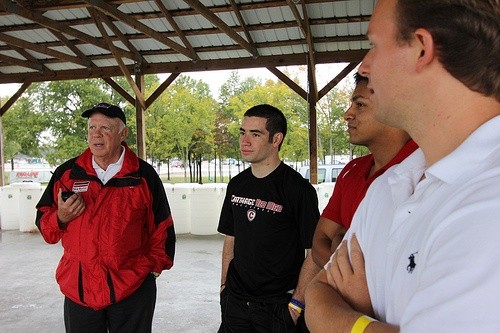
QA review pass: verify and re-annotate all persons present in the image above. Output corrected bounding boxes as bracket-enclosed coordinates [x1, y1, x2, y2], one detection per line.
[216, 104, 319, 333]
[312, 72, 419, 270]
[35, 103, 177, 333]
[305, 1, 500, 333]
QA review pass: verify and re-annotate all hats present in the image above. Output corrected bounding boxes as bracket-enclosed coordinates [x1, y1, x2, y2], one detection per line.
[81, 103, 126, 126]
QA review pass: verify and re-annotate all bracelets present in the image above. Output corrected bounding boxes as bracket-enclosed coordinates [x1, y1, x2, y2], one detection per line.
[288, 300, 305, 316]
[153, 271, 161, 277]
[351, 314, 377, 333]
[220, 284, 225, 288]
